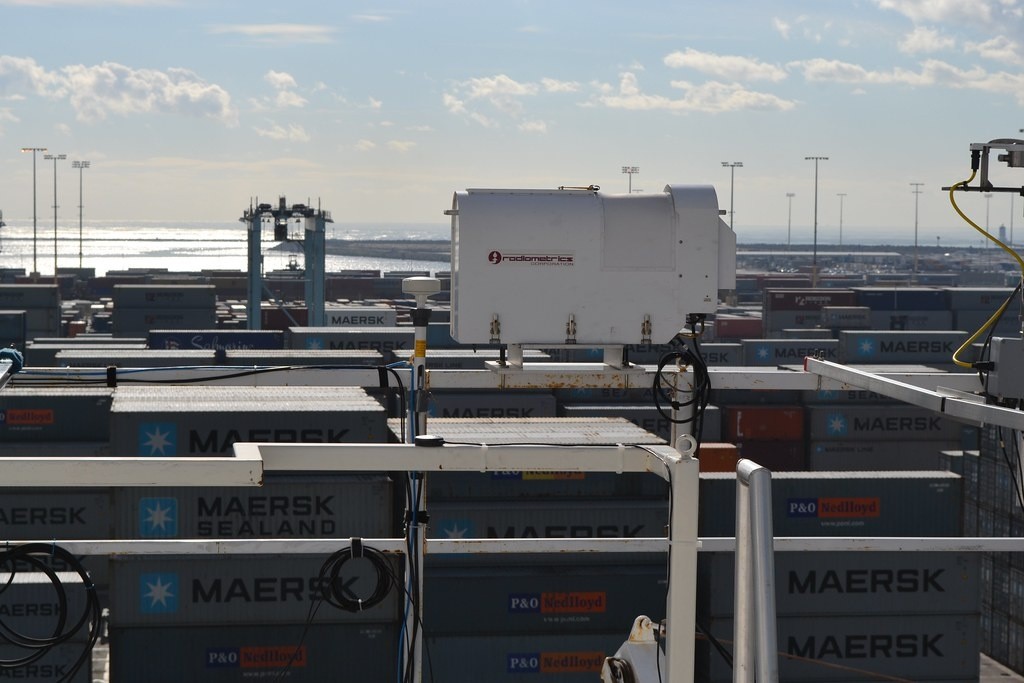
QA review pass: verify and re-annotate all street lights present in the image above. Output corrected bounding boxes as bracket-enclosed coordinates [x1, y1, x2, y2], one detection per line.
[622, 166, 640, 194]
[804, 155, 829, 289]
[786, 192, 797, 252]
[910, 182, 926, 261]
[721, 161, 744, 232]
[43, 154, 67, 285]
[21, 147, 48, 275]
[836, 193, 848, 246]
[984, 193, 994, 250]
[72, 160, 90, 268]
